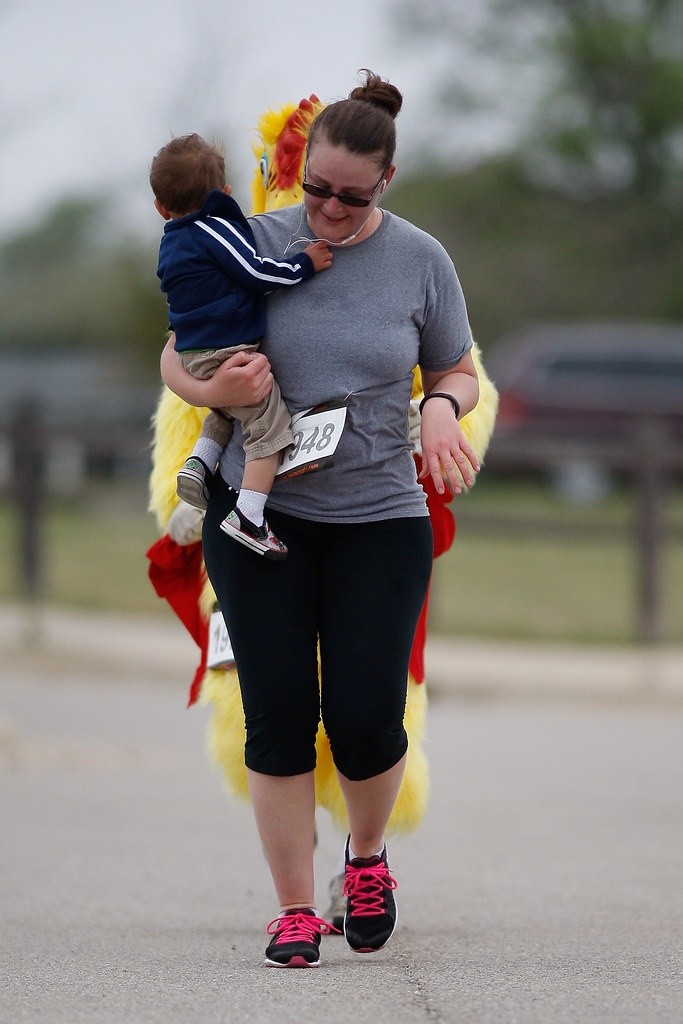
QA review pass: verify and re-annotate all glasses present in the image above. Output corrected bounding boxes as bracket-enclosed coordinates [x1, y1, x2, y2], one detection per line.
[299, 160, 387, 208]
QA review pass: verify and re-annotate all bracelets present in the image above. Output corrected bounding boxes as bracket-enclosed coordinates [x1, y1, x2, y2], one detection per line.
[419, 392, 461, 419]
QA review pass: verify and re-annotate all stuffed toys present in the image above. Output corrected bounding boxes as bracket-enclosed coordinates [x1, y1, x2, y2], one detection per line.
[146, 89, 500, 936]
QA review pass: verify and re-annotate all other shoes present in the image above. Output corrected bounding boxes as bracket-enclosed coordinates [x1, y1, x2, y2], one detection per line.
[324, 873, 346, 934]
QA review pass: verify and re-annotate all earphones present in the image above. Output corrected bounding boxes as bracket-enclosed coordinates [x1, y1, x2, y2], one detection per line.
[383, 179, 386, 190]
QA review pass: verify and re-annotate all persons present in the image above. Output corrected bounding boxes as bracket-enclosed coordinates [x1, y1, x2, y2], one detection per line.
[160, 66, 483, 966]
[150, 130, 334, 562]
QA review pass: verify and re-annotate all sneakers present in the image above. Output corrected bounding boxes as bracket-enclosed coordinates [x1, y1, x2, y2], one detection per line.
[340, 836, 398, 955]
[220, 506, 290, 560]
[266, 907, 342, 967]
[176, 457, 210, 509]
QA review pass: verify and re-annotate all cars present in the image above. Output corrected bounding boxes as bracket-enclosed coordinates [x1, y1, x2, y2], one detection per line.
[478, 320, 683, 489]
